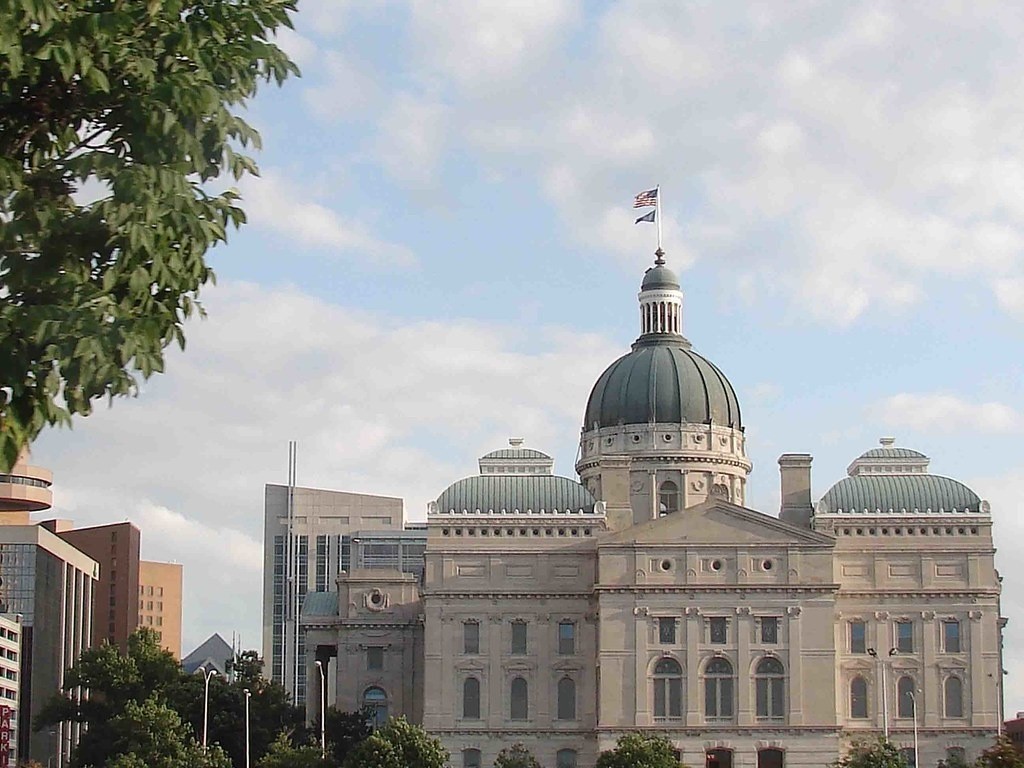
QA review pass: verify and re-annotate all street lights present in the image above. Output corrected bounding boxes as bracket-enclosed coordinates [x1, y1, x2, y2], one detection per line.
[986, 669, 1009, 744]
[243, 688, 253, 768]
[905, 689, 922, 768]
[866, 647, 899, 742]
[197, 666, 219, 755]
[314, 660, 325, 763]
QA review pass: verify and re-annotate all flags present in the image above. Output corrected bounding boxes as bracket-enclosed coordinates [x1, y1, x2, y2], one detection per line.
[633, 188, 657, 206]
[635, 209, 655, 225]
